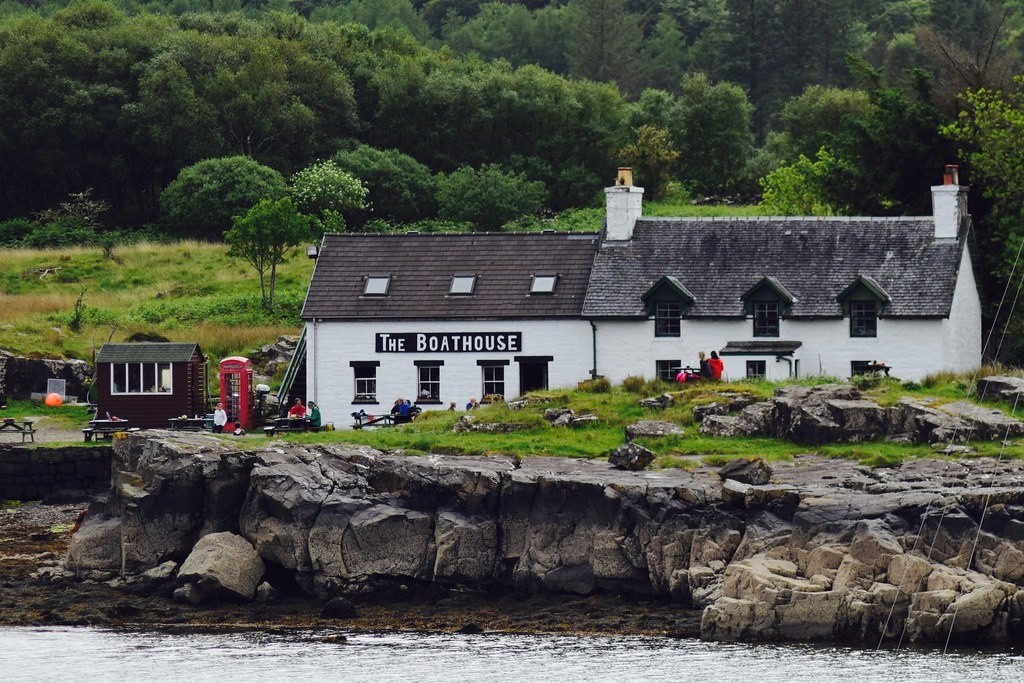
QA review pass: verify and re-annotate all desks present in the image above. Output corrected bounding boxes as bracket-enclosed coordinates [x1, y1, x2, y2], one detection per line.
[0, 421, 35, 444]
[671, 367, 701, 378]
[275, 418, 309, 433]
[351, 413, 395, 429]
[169, 418, 215, 432]
[856, 364, 892, 376]
[88, 420, 128, 437]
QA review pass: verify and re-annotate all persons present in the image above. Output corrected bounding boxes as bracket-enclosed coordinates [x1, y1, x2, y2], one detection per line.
[466, 397, 480, 412]
[391, 399, 413, 425]
[289, 398, 306, 427]
[699, 351, 723, 383]
[493, 397, 498, 405]
[233, 422, 244, 437]
[448, 402, 456, 411]
[304, 401, 321, 427]
[212, 403, 227, 434]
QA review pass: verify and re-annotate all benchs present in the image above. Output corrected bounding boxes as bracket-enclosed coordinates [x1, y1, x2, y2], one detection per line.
[312, 426, 322, 433]
[167, 428, 200, 432]
[274, 428, 303, 434]
[0, 428, 38, 435]
[263, 426, 275, 437]
[95, 428, 140, 442]
[349, 423, 395, 430]
[82, 429, 122, 442]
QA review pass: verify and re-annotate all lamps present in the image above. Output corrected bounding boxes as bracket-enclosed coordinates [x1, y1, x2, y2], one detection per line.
[306, 245, 318, 261]
